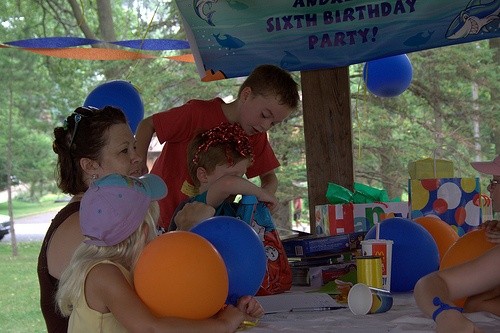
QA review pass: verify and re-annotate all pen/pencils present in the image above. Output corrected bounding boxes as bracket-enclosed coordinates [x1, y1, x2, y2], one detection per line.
[290, 308, 332, 311]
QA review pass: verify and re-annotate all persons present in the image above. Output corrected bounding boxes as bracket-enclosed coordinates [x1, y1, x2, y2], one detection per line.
[136, 64, 301, 233]
[168, 120, 281, 234]
[413, 155, 500, 333]
[37, 105, 215, 333]
[293, 198, 306, 231]
[57, 174, 264, 333]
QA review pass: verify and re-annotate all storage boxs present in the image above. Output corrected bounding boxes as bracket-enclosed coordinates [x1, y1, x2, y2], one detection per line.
[282, 159, 479, 289]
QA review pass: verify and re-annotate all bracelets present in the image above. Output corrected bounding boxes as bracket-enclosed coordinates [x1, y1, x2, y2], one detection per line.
[432, 296, 464, 322]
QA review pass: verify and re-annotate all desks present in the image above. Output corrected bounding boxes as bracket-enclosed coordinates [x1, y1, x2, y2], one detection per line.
[242, 281, 500, 333]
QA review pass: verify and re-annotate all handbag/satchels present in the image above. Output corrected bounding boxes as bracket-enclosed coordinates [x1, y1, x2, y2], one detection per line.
[236, 195, 292, 296]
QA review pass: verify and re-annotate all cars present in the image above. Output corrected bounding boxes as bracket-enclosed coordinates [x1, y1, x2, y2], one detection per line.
[0, 214, 12, 240]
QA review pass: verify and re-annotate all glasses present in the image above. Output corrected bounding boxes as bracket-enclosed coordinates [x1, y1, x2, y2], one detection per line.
[67, 106, 100, 150]
[490, 178, 500, 189]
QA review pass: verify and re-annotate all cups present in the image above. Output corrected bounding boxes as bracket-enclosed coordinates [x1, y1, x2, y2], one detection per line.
[360, 239, 394, 289]
[355, 256, 383, 291]
[348, 282, 393, 315]
[309, 267, 322, 290]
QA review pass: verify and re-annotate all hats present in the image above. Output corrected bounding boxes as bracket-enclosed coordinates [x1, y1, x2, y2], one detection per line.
[79, 173, 168, 246]
[471, 154, 500, 176]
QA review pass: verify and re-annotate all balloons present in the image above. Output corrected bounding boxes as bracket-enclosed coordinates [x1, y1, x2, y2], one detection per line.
[83, 80, 145, 135]
[415, 214, 460, 268]
[439, 226, 497, 308]
[363, 54, 412, 97]
[190, 216, 267, 306]
[364, 217, 440, 293]
[134, 230, 228, 319]
